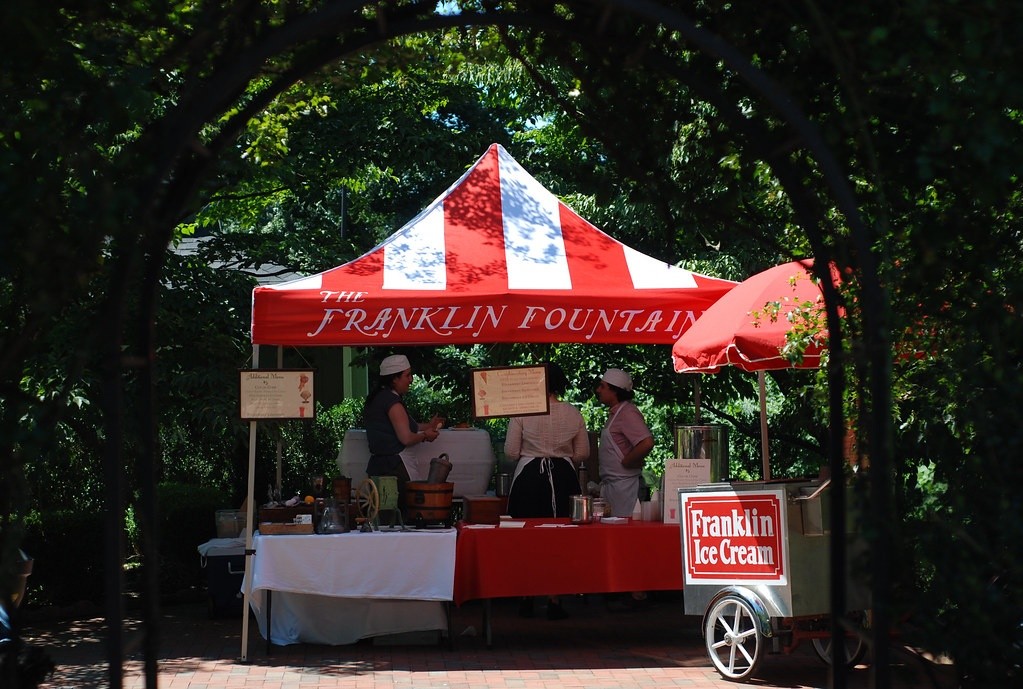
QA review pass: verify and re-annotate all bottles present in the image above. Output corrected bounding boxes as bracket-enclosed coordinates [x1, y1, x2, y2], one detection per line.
[578, 461, 588, 495]
[489, 464, 499, 490]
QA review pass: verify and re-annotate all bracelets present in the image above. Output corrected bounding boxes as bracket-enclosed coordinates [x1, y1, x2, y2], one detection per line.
[422, 432, 427, 442]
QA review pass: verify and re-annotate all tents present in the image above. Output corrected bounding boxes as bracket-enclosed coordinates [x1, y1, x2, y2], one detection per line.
[242, 140, 770, 665]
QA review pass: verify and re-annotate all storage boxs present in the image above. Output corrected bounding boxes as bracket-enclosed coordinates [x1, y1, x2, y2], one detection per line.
[214, 509, 247, 538]
[257, 499, 351, 535]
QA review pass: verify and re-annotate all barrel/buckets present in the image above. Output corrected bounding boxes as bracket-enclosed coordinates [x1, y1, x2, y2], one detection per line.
[673, 422, 729, 482]
[426, 453, 453, 483]
[404, 480, 454, 528]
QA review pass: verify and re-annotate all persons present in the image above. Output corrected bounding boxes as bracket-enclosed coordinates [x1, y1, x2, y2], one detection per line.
[503, 362, 591, 519]
[595, 368, 654, 518]
[362, 355, 446, 525]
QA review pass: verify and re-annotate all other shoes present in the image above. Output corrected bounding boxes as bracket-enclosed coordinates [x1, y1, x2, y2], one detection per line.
[546, 602, 568, 616]
[607, 595, 644, 612]
[519, 606, 528, 617]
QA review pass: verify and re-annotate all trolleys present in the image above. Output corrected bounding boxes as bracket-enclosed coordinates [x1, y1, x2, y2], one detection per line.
[678, 471, 878, 678]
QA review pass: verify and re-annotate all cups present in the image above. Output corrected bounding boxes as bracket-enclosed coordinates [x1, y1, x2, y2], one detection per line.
[567, 494, 593, 524]
[496, 473, 511, 497]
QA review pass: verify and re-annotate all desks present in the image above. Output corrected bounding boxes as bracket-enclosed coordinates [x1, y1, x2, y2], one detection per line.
[242, 526, 457, 647]
[198, 537, 245, 579]
[454, 517, 684, 648]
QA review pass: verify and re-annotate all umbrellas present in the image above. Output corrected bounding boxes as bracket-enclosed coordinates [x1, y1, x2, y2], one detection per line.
[672, 257, 953, 475]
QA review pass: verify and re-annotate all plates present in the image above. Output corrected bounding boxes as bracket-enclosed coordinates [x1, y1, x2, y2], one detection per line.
[406, 525, 456, 533]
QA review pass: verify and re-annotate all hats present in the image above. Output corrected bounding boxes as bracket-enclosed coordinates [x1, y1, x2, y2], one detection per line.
[602, 368, 632, 391]
[380, 355, 410, 375]
